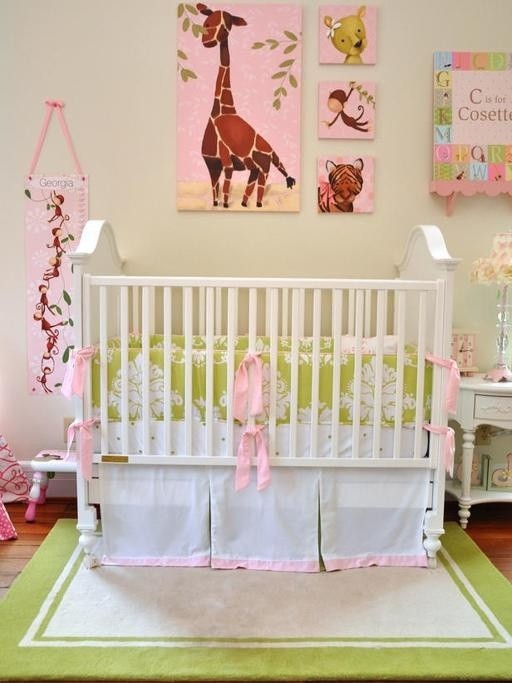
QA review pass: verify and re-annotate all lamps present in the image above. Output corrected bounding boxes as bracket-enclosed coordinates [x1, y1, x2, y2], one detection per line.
[471, 231, 512, 382]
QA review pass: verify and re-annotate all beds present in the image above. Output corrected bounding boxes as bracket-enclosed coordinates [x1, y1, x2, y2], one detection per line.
[65, 217, 463, 573]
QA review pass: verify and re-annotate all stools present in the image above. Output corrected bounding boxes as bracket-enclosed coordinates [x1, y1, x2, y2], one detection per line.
[25, 450, 76, 523]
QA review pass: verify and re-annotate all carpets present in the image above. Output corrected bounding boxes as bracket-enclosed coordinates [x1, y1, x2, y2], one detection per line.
[0, 515, 512, 683]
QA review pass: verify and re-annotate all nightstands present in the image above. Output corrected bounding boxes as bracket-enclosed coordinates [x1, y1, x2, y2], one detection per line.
[451, 375, 511, 530]
